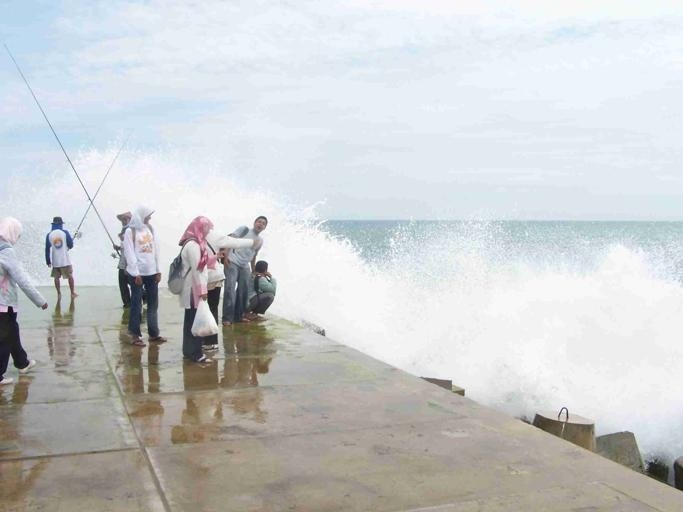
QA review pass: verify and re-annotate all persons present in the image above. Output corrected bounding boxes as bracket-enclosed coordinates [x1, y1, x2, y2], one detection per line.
[221, 215, 268, 327]
[43, 215, 79, 301]
[123, 207, 166, 348]
[249, 258, 277, 320]
[178, 215, 226, 364]
[0, 217, 49, 386]
[112, 211, 147, 308]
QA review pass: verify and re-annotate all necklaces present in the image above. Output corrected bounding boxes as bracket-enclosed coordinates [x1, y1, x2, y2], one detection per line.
[200, 230, 263, 352]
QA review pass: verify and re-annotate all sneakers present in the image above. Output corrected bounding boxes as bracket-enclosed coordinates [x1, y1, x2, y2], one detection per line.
[0, 377, 13, 383]
[19, 359, 36, 373]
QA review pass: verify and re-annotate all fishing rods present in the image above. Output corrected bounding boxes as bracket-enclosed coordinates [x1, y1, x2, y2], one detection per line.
[5, 44, 128, 258]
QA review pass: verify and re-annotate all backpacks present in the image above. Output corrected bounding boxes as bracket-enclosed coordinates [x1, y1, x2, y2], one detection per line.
[168, 239, 202, 294]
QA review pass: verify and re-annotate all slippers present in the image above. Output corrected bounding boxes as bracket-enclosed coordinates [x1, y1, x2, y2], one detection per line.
[149, 337, 166, 341]
[130, 340, 145, 346]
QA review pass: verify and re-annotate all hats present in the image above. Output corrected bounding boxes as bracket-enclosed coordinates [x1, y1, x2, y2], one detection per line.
[51, 217, 66, 224]
